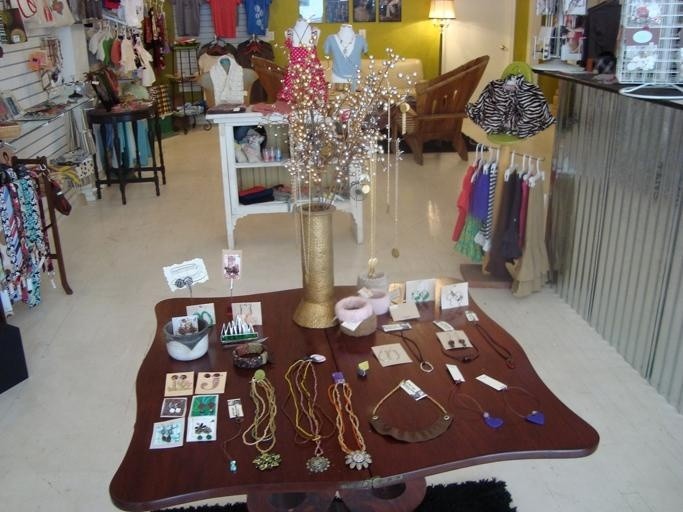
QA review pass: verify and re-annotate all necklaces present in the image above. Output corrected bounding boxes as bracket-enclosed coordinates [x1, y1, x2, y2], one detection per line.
[336, 33, 354, 54]
[293, 25, 309, 48]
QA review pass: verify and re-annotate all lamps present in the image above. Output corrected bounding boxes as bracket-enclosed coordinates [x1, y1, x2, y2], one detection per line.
[428, 1, 456, 75]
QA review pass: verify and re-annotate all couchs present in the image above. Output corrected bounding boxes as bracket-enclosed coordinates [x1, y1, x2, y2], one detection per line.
[282, 58, 425, 135]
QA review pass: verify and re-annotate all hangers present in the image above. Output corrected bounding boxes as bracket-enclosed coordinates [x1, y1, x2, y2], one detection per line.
[246, 36, 262, 56]
[468, 142, 549, 189]
[209, 36, 227, 47]
[144, 1, 167, 16]
[96, 16, 144, 42]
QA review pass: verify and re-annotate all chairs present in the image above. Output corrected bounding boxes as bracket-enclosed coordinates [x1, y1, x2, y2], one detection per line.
[248, 55, 293, 105]
[488, 60, 533, 144]
[391, 55, 488, 167]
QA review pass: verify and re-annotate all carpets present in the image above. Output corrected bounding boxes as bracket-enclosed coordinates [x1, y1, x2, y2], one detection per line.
[377, 130, 490, 154]
[150, 474, 517, 512]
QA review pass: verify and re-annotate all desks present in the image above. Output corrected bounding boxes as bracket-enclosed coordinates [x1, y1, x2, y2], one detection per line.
[107, 274, 600, 512]
[86, 99, 167, 205]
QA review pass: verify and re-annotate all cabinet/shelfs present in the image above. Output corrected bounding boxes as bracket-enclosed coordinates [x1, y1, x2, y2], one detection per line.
[171, 43, 208, 135]
[204, 100, 365, 247]
